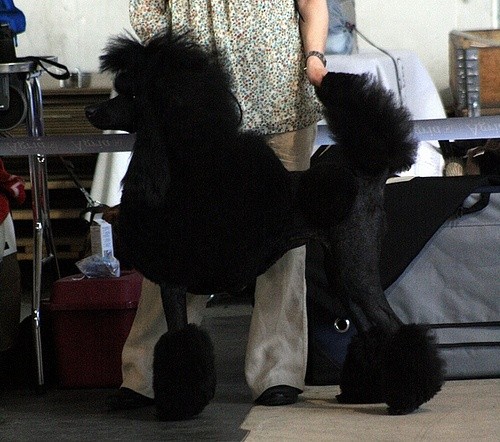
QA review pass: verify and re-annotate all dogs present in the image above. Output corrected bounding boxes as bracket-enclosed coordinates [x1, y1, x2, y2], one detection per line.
[82, 23, 445, 421]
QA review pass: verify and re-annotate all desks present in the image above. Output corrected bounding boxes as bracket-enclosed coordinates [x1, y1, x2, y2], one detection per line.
[85, 52, 446, 223]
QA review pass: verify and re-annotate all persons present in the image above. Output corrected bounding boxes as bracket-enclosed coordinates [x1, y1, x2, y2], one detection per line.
[116, 0, 329, 407]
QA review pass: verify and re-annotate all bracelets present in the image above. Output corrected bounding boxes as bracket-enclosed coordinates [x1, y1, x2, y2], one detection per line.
[304, 51, 326, 68]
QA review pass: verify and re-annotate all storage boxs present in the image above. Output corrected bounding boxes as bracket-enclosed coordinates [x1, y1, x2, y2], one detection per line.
[41, 271, 142, 389]
[90, 218, 114, 259]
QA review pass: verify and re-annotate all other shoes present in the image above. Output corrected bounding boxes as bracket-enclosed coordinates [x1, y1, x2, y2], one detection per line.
[257, 386, 299, 406]
[104, 388, 152, 415]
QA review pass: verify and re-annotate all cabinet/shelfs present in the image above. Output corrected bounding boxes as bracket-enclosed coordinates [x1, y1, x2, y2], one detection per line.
[0, 87, 112, 261]
